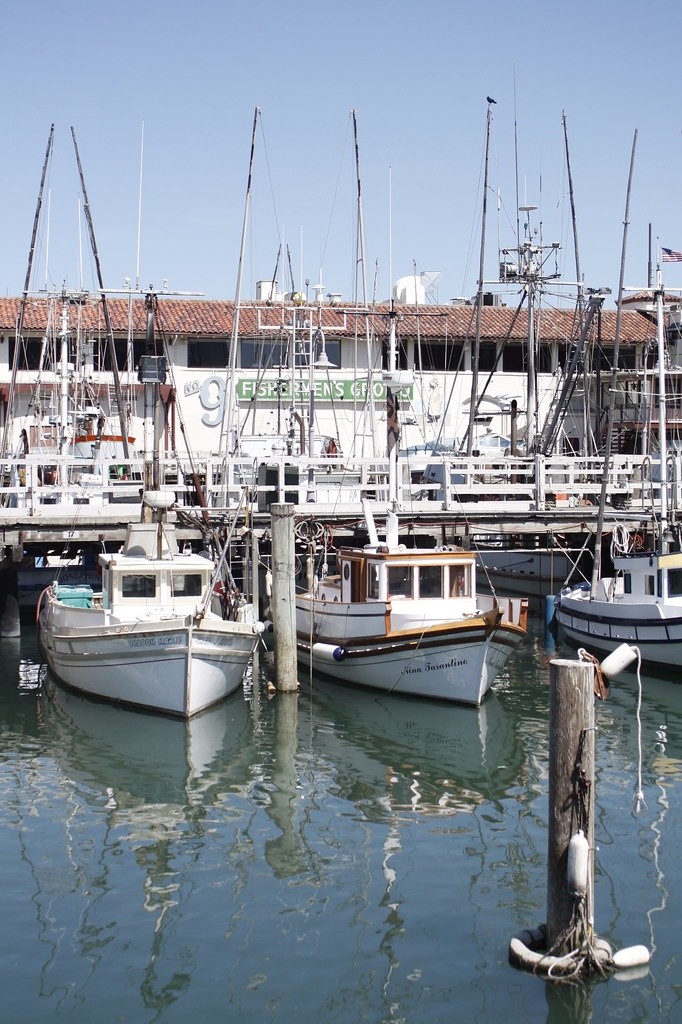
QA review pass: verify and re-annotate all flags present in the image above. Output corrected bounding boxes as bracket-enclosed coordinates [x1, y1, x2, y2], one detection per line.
[660, 247, 682, 262]
[386, 387, 400, 459]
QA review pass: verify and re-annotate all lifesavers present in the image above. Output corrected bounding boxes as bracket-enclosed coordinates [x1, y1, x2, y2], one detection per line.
[629, 535, 643, 550]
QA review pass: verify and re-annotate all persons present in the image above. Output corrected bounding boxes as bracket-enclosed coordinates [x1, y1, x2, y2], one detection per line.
[322, 439, 337, 475]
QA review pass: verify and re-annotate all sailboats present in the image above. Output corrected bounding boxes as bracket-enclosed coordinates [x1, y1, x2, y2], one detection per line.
[0, 64, 682, 716]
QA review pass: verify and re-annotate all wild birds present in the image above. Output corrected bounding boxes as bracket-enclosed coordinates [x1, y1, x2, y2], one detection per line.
[486, 96, 497, 104]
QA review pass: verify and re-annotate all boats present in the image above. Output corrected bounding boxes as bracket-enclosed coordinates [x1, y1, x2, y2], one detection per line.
[292, 659, 529, 795]
[32, 668, 252, 798]
